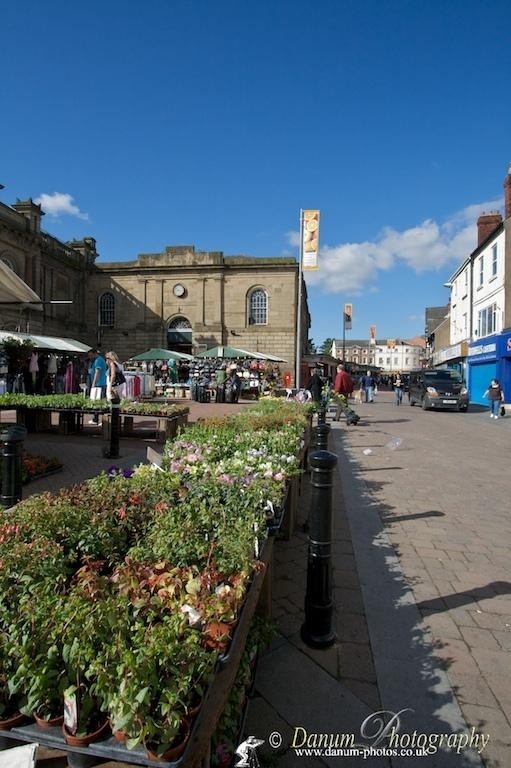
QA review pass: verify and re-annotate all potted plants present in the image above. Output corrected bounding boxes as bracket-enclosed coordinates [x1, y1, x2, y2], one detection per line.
[0, 594, 242, 748]
[0, 337, 36, 371]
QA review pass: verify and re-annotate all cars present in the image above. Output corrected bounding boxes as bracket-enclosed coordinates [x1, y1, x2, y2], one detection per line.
[391, 373, 410, 392]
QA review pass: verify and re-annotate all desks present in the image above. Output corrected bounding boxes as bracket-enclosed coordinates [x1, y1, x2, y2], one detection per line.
[155, 383, 259, 400]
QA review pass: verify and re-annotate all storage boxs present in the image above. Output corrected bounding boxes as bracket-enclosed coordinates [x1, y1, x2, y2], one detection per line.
[166, 388, 190, 398]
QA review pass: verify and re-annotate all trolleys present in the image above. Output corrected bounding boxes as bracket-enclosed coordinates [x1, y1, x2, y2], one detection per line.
[327, 391, 361, 427]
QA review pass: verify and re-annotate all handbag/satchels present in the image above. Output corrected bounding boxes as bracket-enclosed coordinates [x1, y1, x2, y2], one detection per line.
[109, 362, 126, 387]
[500, 406, 506, 417]
[358, 389, 366, 403]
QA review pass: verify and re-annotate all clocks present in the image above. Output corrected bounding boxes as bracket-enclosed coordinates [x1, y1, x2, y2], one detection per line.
[173, 283, 185, 297]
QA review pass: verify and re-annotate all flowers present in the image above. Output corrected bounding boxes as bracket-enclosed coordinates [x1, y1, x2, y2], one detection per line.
[133, 430, 303, 483]
[22, 454, 57, 481]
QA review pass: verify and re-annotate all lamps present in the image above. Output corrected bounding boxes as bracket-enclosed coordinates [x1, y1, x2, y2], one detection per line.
[443, 283, 452, 288]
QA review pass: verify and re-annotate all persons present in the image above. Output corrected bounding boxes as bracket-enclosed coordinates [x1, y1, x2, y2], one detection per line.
[360, 370, 375, 402]
[482, 378, 505, 419]
[87, 349, 107, 426]
[305, 368, 324, 401]
[214, 365, 276, 403]
[392, 374, 404, 406]
[332, 364, 353, 421]
[105, 351, 127, 400]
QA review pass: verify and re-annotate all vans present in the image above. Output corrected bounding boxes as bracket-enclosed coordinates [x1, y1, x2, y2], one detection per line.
[407, 367, 470, 411]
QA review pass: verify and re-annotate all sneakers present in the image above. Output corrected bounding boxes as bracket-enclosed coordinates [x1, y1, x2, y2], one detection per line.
[87, 419, 98, 425]
[490, 413, 499, 419]
